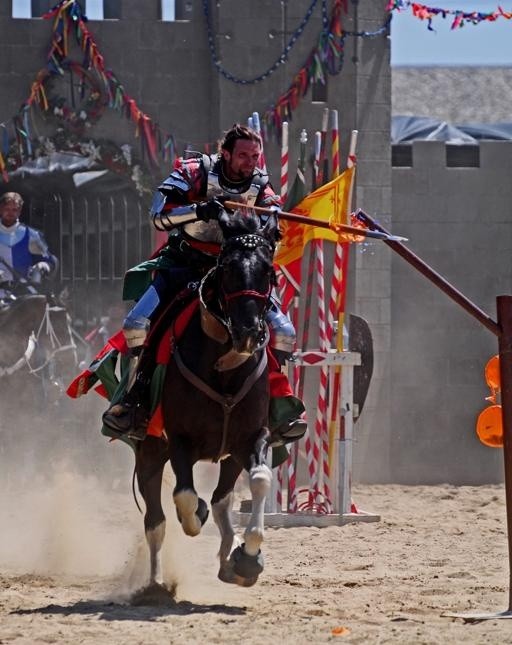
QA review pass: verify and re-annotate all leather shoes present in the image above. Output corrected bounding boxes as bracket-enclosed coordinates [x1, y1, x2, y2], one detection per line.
[102, 401, 135, 435]
[280, 417, 308, 438]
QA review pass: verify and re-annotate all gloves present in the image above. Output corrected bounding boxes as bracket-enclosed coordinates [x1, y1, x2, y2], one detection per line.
[196, 196, 233, 223]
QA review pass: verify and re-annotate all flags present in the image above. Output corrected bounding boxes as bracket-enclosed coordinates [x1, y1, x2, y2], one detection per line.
[273, 166, 353, 266]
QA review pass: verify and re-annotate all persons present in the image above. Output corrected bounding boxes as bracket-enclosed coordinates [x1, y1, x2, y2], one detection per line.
[86, 294, 132, 365]
[0, 192, 61, 296]
[101, 125, 307, 440]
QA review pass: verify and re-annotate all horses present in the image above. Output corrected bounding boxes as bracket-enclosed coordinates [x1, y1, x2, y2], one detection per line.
[0, 286, 80, 493]
[133, 209, 287, 595]
